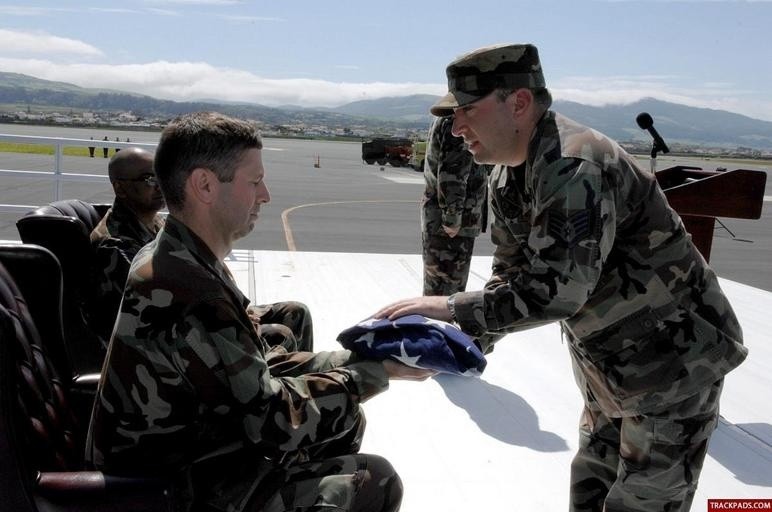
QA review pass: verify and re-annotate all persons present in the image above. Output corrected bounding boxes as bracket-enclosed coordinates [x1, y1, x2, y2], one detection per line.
[88, 136, 95, 158]
[115, 137, 121, 152]
[376, 43, 748, 512]
[422, 115, 489, 296]
[85, 147, 314, 354]
[126, 137, 130, 142]
[82, 111, 443, 512]
[103, 136, 109, 158]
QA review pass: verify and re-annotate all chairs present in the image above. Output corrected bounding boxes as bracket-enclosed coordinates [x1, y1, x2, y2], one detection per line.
[0, 244, 174, 512]
[15, 199, 113, 370]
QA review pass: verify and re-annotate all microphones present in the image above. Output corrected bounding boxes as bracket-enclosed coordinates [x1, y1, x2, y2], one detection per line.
[636, 112, 670, 154]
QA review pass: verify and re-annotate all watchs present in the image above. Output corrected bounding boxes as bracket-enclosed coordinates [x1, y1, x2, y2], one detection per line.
[446, 292, 458, 321]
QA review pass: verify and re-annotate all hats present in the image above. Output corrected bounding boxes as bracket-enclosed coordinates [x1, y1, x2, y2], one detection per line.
[430, 44, 547, 117]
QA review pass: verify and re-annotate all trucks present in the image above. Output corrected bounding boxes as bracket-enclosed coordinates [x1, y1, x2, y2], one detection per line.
[412, 142, 427, 171]
[363, 137, 411, 172]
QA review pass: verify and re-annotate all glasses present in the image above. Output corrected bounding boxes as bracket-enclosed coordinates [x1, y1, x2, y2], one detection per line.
[115, 174, 160, 187]
